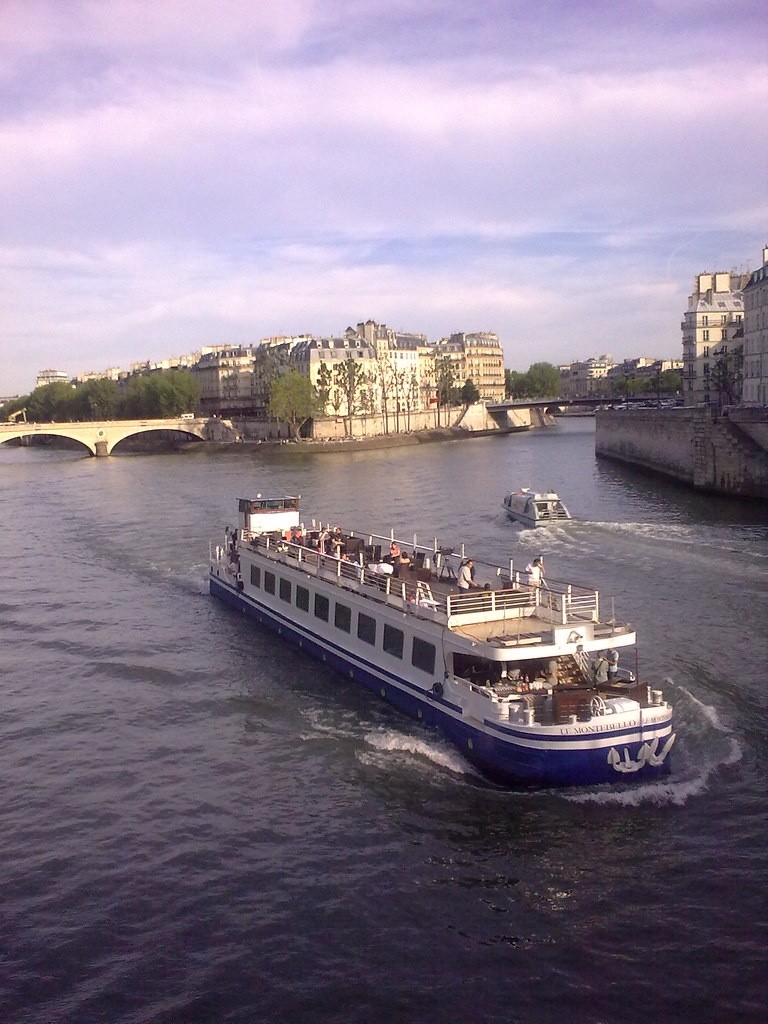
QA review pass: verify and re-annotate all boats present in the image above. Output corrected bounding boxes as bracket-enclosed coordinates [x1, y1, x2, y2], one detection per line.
[500, 488, 573, 528]
[209, 494, 677, 792]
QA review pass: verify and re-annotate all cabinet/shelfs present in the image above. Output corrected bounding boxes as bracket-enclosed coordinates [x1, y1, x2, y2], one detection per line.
[552, 683, 597, 725]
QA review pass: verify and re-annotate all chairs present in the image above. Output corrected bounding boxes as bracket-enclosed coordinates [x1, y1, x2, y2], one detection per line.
[256, 531, 364, 567]
[378, 573, 393, 591]
[412, 559, 431, 580]
[416, 581, 440, 611]
[384, 554, 392, 564]
[395, 563, 411, 580]
[413, 552, 425, 569]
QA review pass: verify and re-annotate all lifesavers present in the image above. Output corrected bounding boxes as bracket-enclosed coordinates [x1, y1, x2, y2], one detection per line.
[432, 682, 443, 698]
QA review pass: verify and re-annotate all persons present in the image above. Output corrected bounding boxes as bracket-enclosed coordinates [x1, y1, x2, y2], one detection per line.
[477, 583, 492, 611]
[591, 648, 619, 688]
[290, 531, 303, 558]
[407, 595, 415, 603]
[376, 541, 411, 585]
[316, 526, 359, 565]
[506, 671, 532, 691]
[456, 558, 478, 612]
[525, 559, 545, 606]
